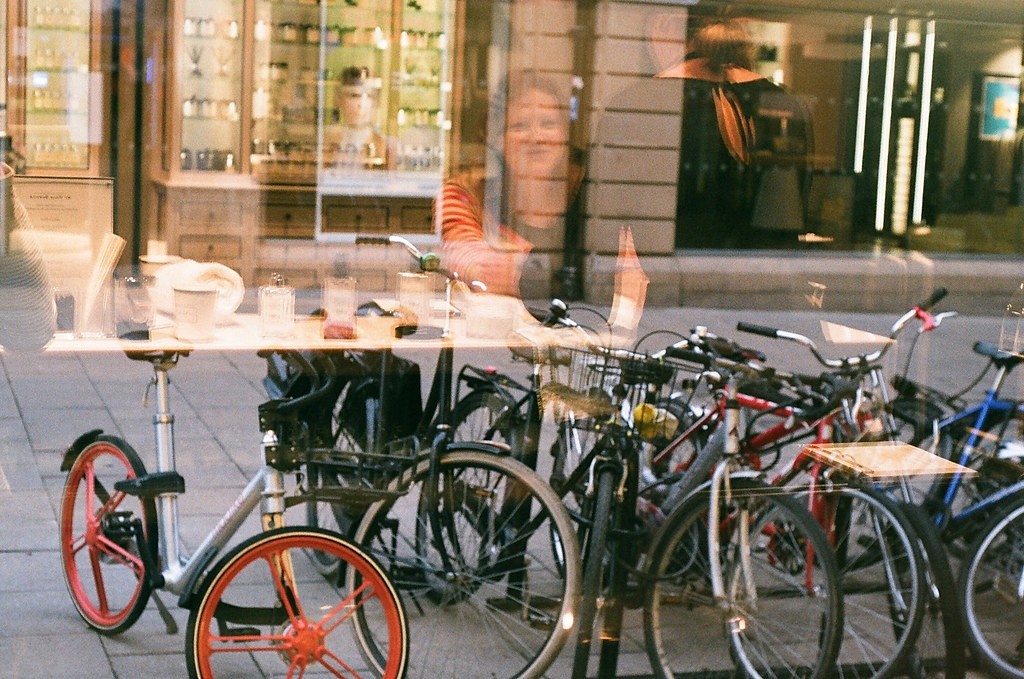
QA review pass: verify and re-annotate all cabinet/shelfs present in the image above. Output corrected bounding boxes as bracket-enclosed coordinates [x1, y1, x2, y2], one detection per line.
[6, 0, 450, 238]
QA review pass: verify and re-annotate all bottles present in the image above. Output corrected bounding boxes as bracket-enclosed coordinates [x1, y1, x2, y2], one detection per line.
[321, 262, 358, 340]
[396, 258, 437, 327]
[181, 17, 444, 172]
[259, 274, 294, 338]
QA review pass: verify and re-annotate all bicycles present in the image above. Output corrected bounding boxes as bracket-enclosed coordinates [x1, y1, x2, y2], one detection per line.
[267, 232, 580, 679]
[59, 302, 409, 679]
[412, 287, 1024, 679]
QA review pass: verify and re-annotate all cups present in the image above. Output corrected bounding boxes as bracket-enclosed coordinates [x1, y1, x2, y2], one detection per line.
[174, 282, 216, 342]
[136, 256, 179, 280]
[74, 286, 112, 342]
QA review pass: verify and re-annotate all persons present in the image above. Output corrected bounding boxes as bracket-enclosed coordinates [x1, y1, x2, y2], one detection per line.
[588, 5, 841, 411]
[433, 66, 647, 351]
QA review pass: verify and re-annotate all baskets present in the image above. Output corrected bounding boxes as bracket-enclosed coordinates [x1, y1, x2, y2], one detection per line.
[302, 421, 419, 505]
[557, 345, 704, 449]
